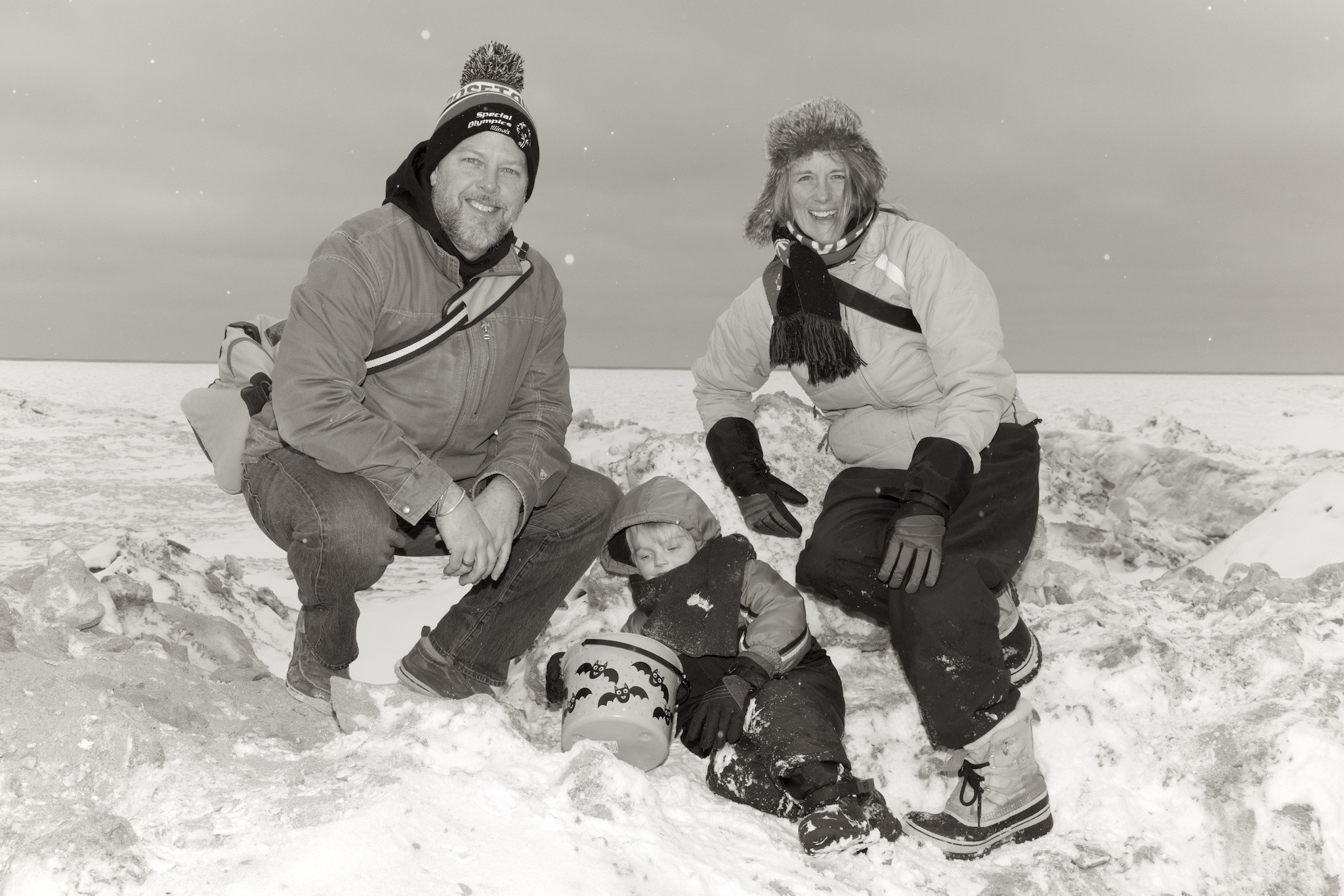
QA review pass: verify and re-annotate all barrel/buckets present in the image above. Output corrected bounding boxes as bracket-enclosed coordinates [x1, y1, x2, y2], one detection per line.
[559, 633, 692, 773]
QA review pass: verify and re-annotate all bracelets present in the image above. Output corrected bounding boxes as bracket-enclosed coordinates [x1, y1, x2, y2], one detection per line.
[429, 488, 447, 518]
[439, 484, 466, 516]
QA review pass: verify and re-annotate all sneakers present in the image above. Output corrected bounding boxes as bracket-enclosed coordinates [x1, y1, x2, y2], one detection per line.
[283, 608, 350, 712]
[393, 627, 496, 701]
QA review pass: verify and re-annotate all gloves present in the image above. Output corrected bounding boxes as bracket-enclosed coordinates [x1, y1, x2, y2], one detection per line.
[686, 657, 769, 755]
[546, 651, 567, 705]
[877, 436, 974, 595]
[706, 417, 809, 537]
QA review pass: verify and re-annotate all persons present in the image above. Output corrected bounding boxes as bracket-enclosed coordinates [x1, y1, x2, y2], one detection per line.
[542, 476, 902, 855]
[243, 44, 623, 715]
[694, 93, 1054, 860]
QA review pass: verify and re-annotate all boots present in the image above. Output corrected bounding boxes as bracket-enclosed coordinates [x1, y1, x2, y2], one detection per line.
[900, 712, 1054, 860]
[987, 578, 1042, 688]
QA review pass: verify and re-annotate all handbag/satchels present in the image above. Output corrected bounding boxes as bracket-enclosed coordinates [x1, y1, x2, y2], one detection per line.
[183, 318, 286, 494]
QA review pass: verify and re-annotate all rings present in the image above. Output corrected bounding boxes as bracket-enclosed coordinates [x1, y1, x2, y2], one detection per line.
[462, 559, 475, 567]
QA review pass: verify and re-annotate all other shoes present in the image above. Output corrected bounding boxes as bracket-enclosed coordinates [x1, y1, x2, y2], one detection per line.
[799, 788, 903, 854]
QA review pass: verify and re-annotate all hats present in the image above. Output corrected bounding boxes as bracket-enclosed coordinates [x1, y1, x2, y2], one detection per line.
[744, 99, 887, 245]
[422, 41, 540, 203]
